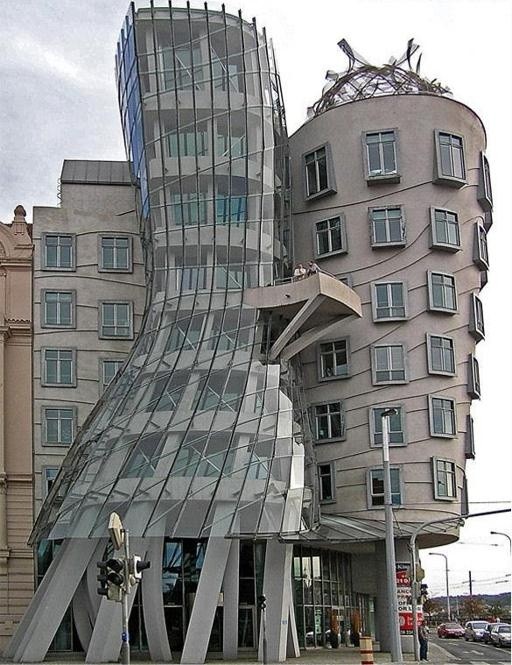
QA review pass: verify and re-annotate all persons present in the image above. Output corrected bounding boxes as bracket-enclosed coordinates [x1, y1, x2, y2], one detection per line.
[419, 619, 431, 662]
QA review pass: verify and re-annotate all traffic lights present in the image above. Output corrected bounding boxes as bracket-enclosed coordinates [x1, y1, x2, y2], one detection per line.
[133, 554, 151, 580]
[418, 582, 428, 599]
[258, 593, 267, 610]
[96, 558, 118, 599]
[107, 555, 125, 589]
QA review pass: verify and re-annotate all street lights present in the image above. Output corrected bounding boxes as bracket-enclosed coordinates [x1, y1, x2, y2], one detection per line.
[490, 531, 511, 557]
[427, 552, 451, 621]
[107, 511, 132, 665]
[379, 408, 406, 663]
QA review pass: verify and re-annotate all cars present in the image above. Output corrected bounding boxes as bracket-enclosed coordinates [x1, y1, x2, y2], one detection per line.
[305, 627, 363, 647]
[435, 620, 510, 650]
